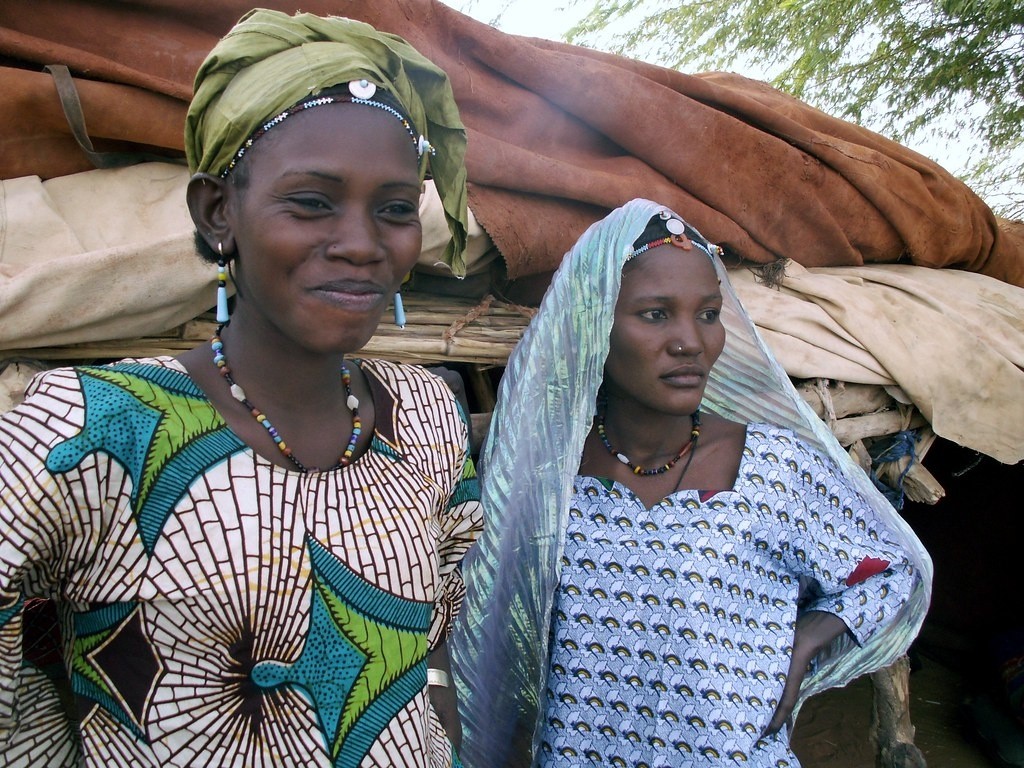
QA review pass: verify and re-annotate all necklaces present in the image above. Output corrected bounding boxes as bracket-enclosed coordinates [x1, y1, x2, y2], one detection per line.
[595, 398, 701, 476]
[208, 320, 368, 474]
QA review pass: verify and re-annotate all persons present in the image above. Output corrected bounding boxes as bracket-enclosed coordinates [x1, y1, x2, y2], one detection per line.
[448, 198, 936, 766]
[0, 4, 492, 768]
